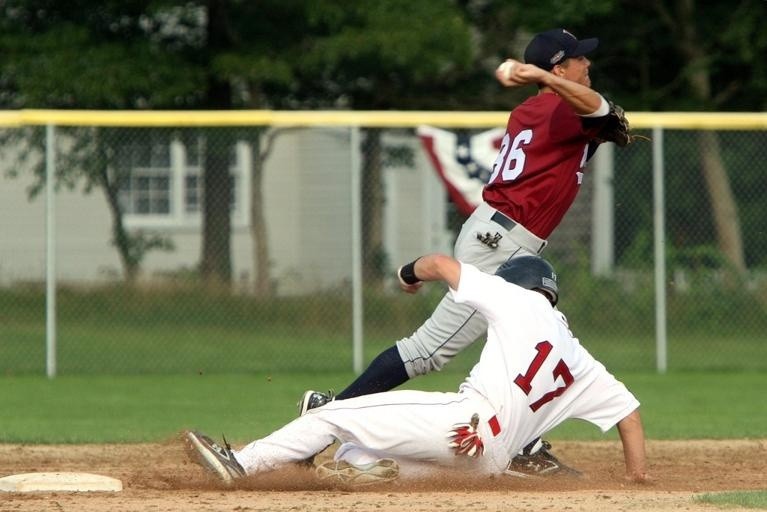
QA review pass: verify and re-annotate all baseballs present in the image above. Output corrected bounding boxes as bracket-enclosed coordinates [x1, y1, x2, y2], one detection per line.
[498, 62, 517, 80]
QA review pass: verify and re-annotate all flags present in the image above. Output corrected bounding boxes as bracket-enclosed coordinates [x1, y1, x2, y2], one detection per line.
[415, 123, 509, 220]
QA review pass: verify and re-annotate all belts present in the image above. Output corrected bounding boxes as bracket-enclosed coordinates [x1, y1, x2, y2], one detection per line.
[491, 211, 517, 231]
[488, 415, 501, 437]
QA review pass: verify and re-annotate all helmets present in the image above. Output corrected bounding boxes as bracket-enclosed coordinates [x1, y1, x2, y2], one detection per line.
[494, 255, 560, 307]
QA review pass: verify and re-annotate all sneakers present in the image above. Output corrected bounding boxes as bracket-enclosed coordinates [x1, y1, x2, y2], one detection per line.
[296, 387, 335, 472]
[313, 458, 400, 489]
[503, 435, 590, 482]
[181, 427, 249, 490]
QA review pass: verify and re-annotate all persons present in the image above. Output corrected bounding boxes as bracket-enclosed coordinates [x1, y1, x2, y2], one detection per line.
[297, 30, 630, 486]
[179, 254, 654, 494]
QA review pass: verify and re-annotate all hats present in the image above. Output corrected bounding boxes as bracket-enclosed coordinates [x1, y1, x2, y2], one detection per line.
[524, 27, 599, 71]
